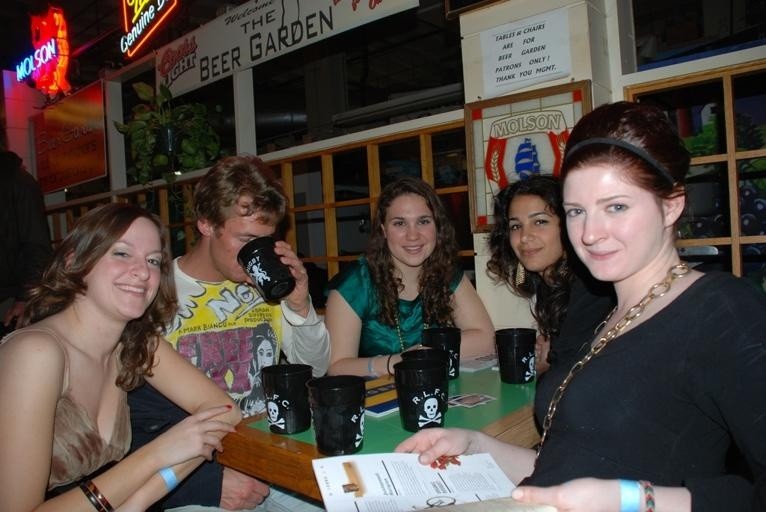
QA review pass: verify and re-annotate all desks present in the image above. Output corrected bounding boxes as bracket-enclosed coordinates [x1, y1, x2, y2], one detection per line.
[218, 368, 544, 506]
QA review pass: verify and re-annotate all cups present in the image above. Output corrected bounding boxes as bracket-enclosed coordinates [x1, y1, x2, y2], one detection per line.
[496, 327, 537, 382]
[308, 374, 366, 453]
[238, 236, 295, 300]
[261, 363, 313, 435]
[393, 326, 461, 433]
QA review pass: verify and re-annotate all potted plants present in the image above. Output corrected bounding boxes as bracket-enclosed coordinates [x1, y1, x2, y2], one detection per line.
[113, 82, 229, 244]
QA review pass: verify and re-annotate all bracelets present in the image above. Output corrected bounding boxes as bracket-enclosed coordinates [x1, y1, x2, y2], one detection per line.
[85, 479, 114, 512]
[78, 484, 107, 512]
[369, 354, 383, 379]
[159, 468, 177, 492]
[640, 478, 656, 512]
[619, 477, 641, 512]
[387, 352, 395, 377]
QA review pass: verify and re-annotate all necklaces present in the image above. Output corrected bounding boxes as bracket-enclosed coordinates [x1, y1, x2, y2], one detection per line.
[396, 313, 430, 351]
[534, 262, 691, 460]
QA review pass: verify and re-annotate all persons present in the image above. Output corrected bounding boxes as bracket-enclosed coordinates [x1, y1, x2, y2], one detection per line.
[0, 126, 51, 331]
[392, 100, 766, 512]
[484, 175, 599, 374]
[128, 154, 333, 510]
[322, 178, 498, 377]
[0, 203, 244, 511]
